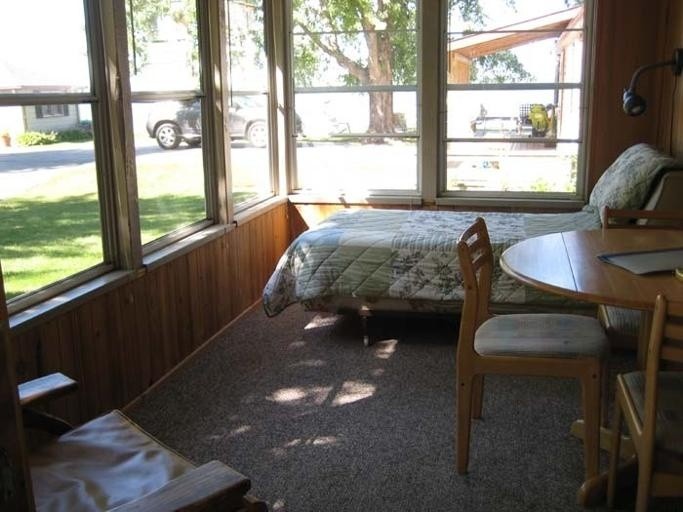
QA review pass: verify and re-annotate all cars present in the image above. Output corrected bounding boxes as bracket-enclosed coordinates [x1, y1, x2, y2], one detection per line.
[146, 87, 301, 148]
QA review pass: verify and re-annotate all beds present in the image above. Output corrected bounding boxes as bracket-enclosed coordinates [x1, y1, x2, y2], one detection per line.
[260, 142, 681, 348]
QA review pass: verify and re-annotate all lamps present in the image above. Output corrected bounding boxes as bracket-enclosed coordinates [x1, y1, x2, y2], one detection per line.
[620, 45, 683, 120]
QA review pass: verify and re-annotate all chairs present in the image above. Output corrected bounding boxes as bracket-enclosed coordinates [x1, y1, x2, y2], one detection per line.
[0, 264, 283, 508]
[599, 203, 683, 424]
[454, 216, 607, 485]
[611, 293, 683, 507]
